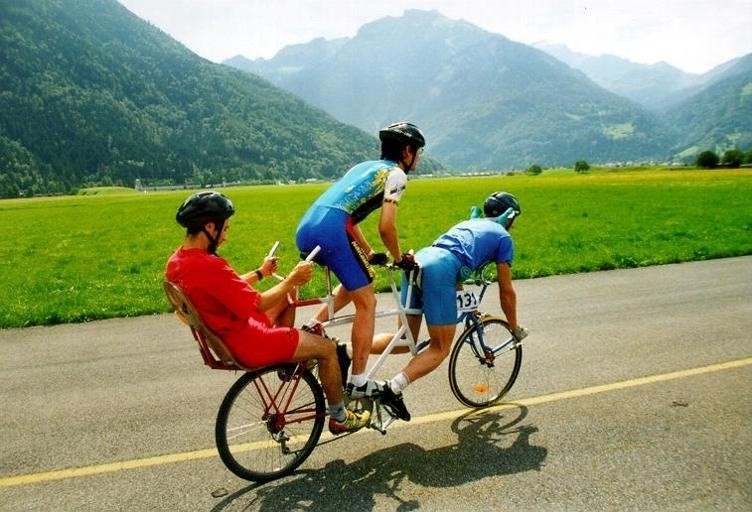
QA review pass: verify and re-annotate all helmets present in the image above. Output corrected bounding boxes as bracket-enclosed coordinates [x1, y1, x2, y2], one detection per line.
[379, 122, 425, 147]
[483, 191, 521, 217]
[176, 191, 235, 227]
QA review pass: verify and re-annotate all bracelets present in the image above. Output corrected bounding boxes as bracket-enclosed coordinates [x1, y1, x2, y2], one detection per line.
[256, 268, 263, 282]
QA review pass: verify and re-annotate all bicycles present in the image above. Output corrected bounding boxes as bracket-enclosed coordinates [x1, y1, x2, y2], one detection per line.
[214, 252, 524, 483]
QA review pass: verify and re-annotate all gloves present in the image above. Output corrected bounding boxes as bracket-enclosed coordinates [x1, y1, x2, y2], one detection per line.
[367, 252, 389, 265]
[511, 328, 529, 346]
[393, 253, 415, 271]
[480, 312, 493, 321]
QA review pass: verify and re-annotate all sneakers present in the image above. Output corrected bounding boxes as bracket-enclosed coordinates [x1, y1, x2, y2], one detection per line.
[277, 324, 410, 422]
[328, 409, 371, 435]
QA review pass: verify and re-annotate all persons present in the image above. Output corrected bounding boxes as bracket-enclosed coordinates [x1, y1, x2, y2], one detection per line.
[162, 190, 374, 436]
[293, 120, 428, 402]
[334, 192, 530, 424]
